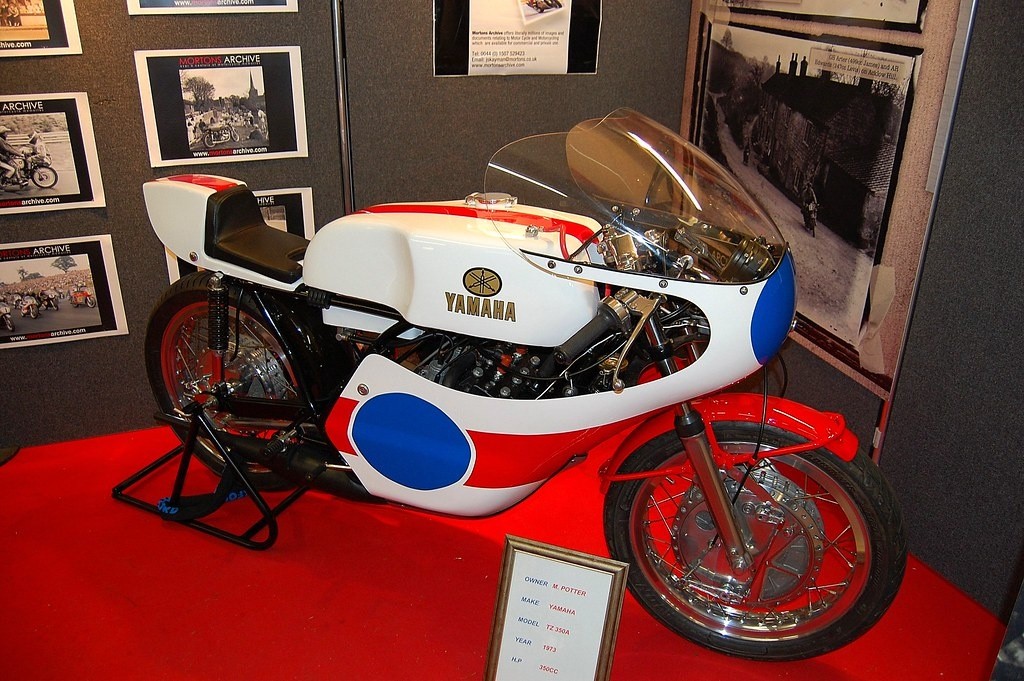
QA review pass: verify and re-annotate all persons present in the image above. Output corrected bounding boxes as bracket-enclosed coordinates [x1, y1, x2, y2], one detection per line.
[186, 106, 267, 143]
[0, 279, 96, 316]
[0, 126, 51, 191]
[743, 142, 751, 163]
[801, 184, 817, 230]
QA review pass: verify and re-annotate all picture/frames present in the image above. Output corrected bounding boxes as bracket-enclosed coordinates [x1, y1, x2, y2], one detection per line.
[133, 46, 309, 168]
[0, 92, 107, 215]
[126, 0, 298, 15]
[0, 0, 83, 57]
[0, 234, 129, 350]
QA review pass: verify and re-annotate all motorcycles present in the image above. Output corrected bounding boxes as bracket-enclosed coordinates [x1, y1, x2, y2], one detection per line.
[201, 120, 240, 147]
[526, 0, 562, 13]
[0, 150, 58, 190]
[108, 106, 908, 664]
[800, 201, 818, 237]
[0, 284, 96, 332]
